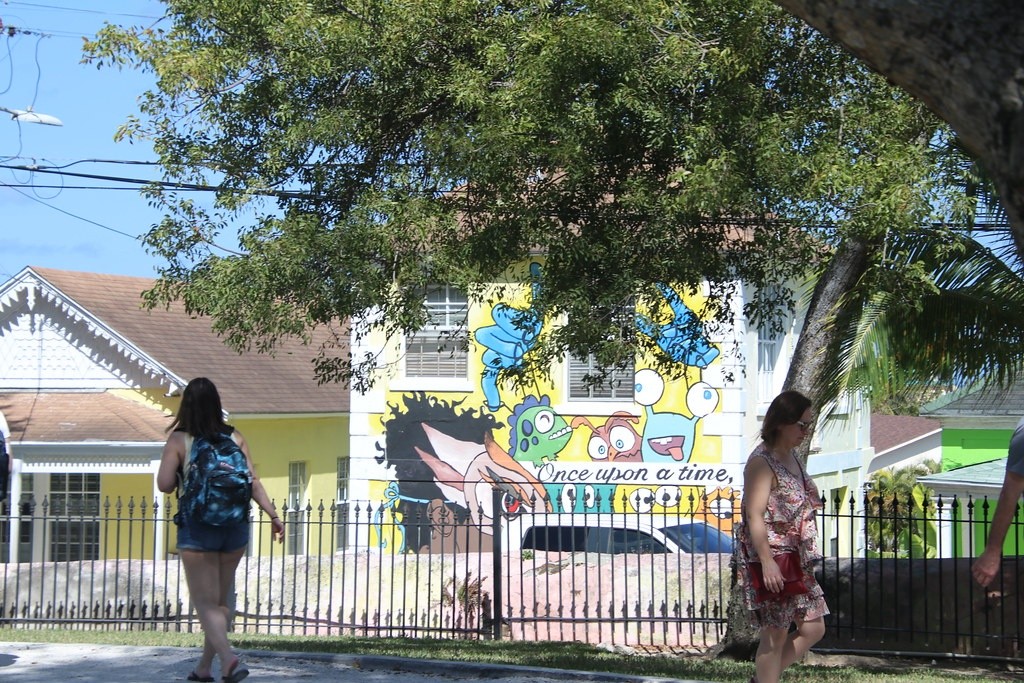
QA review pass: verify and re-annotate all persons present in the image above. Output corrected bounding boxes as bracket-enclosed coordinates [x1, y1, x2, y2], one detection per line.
[730, 389, 827, 683]
[156, 376, 287, 683]
[971, 413, 1024, 588]
[0, 411, 12, 501]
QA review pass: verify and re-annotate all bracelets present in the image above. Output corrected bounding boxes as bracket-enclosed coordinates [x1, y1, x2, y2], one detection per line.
[272, 517, 279, 520]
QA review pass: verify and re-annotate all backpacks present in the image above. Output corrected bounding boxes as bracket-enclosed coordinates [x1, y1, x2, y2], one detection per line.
[174, 425, 251, 529]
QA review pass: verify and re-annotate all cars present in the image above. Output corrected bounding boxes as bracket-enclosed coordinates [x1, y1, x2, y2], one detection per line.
[513, 515, 734, 553]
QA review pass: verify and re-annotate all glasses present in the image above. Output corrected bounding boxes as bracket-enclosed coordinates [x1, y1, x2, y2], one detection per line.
[796, 420, 814, 431]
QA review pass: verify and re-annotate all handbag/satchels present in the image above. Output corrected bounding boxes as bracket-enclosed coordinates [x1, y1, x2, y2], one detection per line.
[746, 550, 810, 603]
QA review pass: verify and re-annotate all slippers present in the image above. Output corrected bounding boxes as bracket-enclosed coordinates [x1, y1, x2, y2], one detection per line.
[187, 670, 213, 680]
[221, 668, 249, 682]
